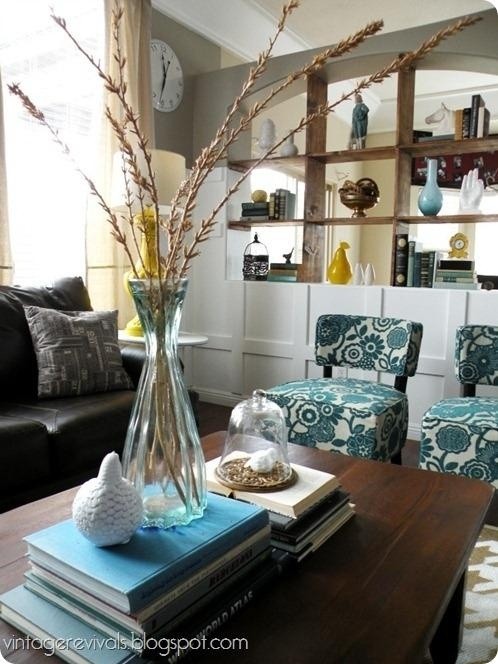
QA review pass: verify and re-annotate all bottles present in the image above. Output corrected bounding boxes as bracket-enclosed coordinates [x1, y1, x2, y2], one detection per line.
[279, 129, 299, 157]
[352, 262, 376, 286]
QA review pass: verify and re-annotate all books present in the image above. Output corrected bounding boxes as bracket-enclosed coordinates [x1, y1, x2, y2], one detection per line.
[394, 235, 482, 290]
[240, 188, 296, 221]
[267, 263, 304, 281]
[418, 94, 489, 143]
[0, 449, 356, 664]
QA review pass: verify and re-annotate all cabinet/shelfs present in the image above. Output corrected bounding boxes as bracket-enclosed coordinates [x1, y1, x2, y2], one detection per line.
[226, 53, 498, 290]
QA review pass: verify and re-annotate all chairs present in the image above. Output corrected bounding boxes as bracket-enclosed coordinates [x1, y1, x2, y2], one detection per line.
[417, 324, 498, 490]
[253, 313, 423, 466]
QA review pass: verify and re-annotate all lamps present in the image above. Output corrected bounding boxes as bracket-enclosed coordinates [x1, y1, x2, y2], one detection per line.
[107, 148, 186, 337]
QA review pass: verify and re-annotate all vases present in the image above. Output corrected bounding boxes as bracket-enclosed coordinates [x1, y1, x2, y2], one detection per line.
[120, 276, 208, 530]
[353, 263, 363, 286]
[281, 132, 298, 156]
[364, 264, 375, 286]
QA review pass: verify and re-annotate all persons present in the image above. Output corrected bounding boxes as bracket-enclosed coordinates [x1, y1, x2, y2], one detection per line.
[347, 93, 369, 150]
[456, 165, 484, 261]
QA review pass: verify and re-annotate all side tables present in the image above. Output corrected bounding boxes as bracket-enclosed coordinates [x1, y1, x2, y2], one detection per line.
[117, 328, 208, 346]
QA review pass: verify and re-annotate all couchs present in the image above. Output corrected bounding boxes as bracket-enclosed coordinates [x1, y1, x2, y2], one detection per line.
[0, 276, 200, 516]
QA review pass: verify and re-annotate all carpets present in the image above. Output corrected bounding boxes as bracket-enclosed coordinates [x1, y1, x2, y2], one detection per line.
[416, 524, 498, 664]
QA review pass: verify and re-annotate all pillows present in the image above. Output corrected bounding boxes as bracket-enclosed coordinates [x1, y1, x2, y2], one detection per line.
[22, 301, 136, 399]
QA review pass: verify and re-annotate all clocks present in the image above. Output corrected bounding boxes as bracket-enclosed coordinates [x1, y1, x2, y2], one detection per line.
[447, 233, 470, 259]
[149, 38, 184, 114]
